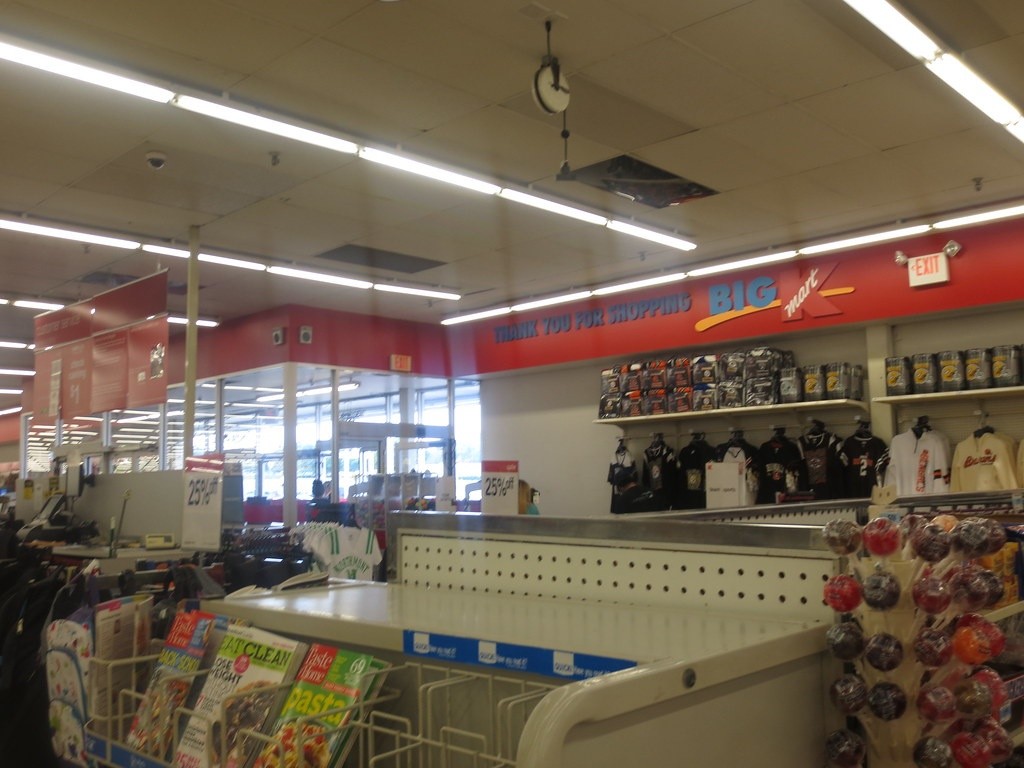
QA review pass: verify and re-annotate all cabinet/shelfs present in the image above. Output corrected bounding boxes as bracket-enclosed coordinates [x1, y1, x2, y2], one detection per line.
[201, 578, 807, 768]
[982, 513, 1024, 764]
[611, 399, 872, 527]
[868, 386, 1024, 519]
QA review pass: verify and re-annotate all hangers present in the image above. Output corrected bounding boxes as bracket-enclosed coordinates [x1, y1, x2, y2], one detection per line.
[311, 502, 362, 530]
[914, 411, 989, 433]
[615, 416, 869, 450]
[241, 533, 287, 555]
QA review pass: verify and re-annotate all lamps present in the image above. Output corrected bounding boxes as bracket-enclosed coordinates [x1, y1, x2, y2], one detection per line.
[0, 0, 1023, 415]
